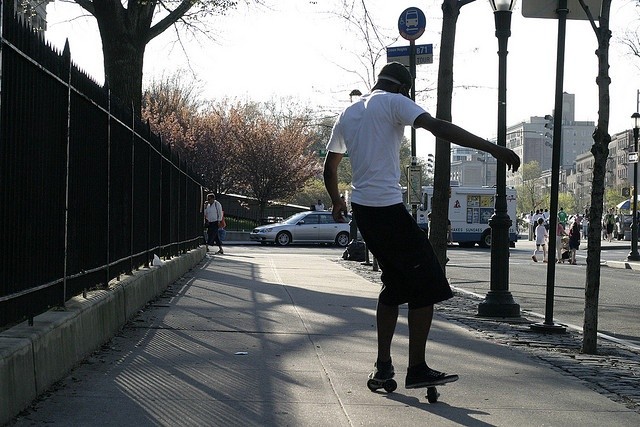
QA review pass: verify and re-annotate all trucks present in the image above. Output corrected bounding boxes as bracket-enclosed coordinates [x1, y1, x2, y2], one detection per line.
[400, 185, 521, 248]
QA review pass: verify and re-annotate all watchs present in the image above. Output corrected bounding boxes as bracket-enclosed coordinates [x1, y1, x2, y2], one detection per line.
[332, 194, 340, 204]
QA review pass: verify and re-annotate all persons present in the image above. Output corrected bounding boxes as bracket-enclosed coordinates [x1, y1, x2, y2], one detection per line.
[218, 211, 226, 240]
[555, 216, 568, 264]
[204, 193, 224, 255]
[532, 209, 546, 240]
[574, 207, 591, 239]
[310, 205, 316, 211]
[323, 61, 520, 390]
[544, 211, 550, 223]
[340, 196, 346, 204]
[569, 222, 580, 265]
[204, 201, 209, 208]
[316, 199, 324, 211]
[531, 218, 548, 263]
[602, 207, 624, 241]
[349, 209, 353, 213]
[543, 209, 549, 219]
[539, 209, 544, 214]
[557, 207, 568, 230]
[528, 210, 533, 241]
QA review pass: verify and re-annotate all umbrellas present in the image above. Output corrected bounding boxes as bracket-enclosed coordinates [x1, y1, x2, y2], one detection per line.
[616, 199, 640, 210]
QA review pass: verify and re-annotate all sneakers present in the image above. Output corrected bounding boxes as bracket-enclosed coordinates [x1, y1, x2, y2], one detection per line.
[371, 358, 394, 381]
[531, 256, 538, 262]
[541, 260, 548, 262]
[406, 361, 459, 389]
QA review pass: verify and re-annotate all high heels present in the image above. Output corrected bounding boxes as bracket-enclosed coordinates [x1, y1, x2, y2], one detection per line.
[215, 249, 223, 253]
[206, 248, 209, 251]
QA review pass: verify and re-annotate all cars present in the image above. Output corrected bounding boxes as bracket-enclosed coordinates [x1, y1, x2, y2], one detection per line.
[249, 210, 362, 247]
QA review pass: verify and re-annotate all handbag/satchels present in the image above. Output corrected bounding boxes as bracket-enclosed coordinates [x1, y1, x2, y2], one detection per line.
[216, 211, 226, 227]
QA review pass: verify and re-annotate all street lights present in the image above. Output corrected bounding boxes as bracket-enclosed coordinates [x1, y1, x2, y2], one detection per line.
[478, 0, 520, 317]
[626, 113, 640, 261]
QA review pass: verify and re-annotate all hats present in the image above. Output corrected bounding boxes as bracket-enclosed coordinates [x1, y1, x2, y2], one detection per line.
[379, 61, 412, 98]
